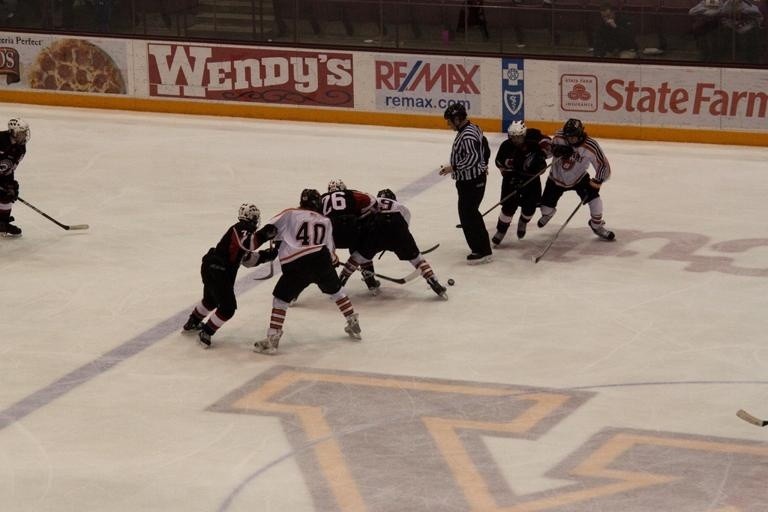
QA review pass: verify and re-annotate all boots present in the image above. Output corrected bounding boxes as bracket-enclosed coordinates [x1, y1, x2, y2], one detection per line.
[427, 277, 446, 296]
[184, 313, 205, 330]
[0, 216, 21, 235]
[538, 209, 557, 228]
[198, 323, 215, 345]
[362, 267, 380, 290]
[517, 216, 531, 238]
[492, 216, 511, 243]
[338, 271, 350, 286]
[344, 314, 361, 334]
[253, 328, 283, 350]
[588, 219, 615, 240]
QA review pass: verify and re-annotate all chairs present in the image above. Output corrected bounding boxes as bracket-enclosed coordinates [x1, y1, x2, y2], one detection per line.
[1, 1, 132, 36]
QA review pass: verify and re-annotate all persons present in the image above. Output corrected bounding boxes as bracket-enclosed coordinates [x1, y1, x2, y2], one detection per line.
[688, 0, 764, 65]
[492, 119, 552, 248]
[289, 181, 380, 307]
[438, 103, 493, 264]
[330, 187, 449, 301]
[538, 119, 615, 241]
[181, 203, 278, 349]
[592, 2, 637, 58]
[0, 118, 32, 237]
[457, 0, 488, 41]
[253, 189, 362, 355]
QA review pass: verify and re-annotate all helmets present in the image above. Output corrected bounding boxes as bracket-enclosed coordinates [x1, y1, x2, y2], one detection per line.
[508, 120, 527, 137]
[564, 119, 583, 143]
[301, 189, 323, 211]
[8, 118, 30, 145]
[377, 189, 396, 201]
[328, 178, 347, 192]
[443, 103, 467, 120]
[237, 204, 260, 225]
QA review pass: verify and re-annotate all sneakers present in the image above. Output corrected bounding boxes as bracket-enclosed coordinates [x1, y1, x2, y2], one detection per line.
[466, 250, 493, 263]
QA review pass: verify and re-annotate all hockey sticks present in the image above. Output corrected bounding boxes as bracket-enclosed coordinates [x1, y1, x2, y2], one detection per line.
[736, 409, 768, 427]
[0, 186, 88, 230]
[456, 155, 563, 228]
[339, 262, 422, 284]
[254, 239, 274, 280]
[535, 195, 588, 263]
[421, 244, 440, 255]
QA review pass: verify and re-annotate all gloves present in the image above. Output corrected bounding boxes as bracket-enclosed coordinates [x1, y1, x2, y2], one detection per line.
[259, 247, 277, 262]
[585, 176, 601, 198]
[2, 185, 19, 203]
[554, 144, 573, 161]
